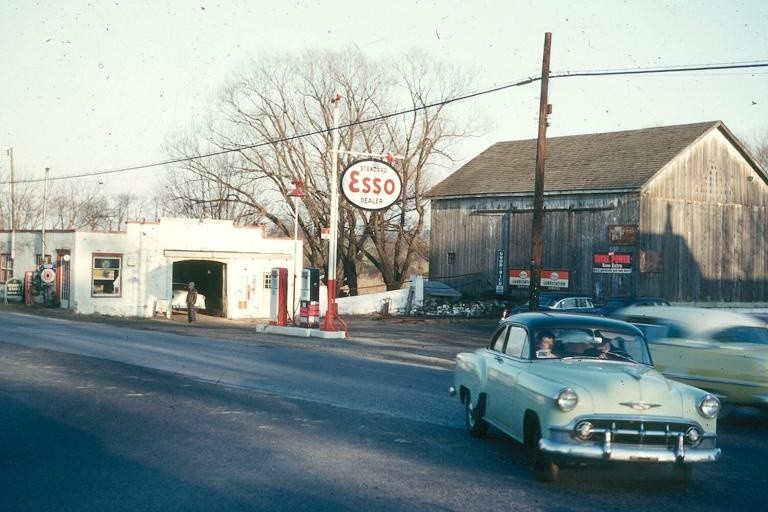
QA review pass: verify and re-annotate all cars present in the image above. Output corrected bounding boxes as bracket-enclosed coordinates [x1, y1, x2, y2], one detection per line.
[172, 281, 207, 312]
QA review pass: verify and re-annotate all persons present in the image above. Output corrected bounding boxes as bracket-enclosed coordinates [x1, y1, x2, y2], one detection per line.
[536, 332, 557, 357]
[186, 282, 197, 322]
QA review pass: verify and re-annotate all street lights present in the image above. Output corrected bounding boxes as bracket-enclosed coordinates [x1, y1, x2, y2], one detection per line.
[40, 166, 51, 262]
[286, 178, 309, 326]
